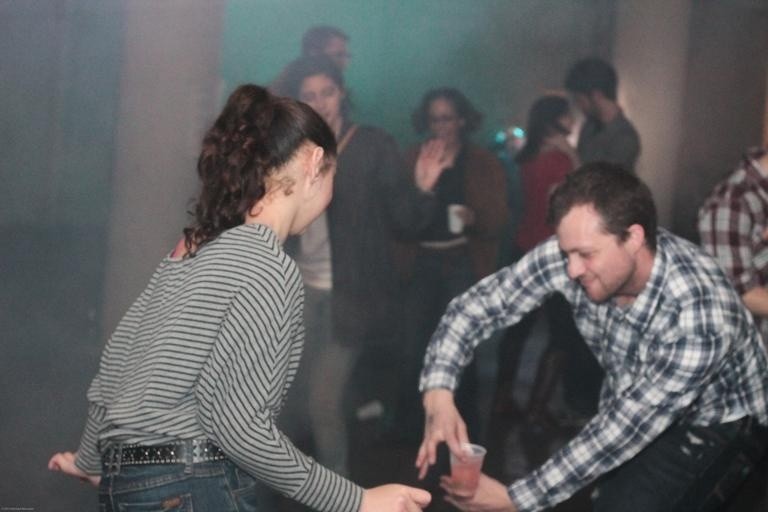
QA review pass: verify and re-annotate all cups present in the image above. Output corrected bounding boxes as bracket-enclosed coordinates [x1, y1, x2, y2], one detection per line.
[450, 444, 485, 498]
[447, 204, 465, 235]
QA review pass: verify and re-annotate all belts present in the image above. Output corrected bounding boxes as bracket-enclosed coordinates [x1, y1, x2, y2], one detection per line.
[104, 438, 228, 467]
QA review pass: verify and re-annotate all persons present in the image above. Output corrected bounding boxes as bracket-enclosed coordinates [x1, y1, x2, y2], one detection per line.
[49, 82, 436, 512]
[698, 149, 767, 342]
[265, 27, 642, 510]
[415, 162, 768, 512]
